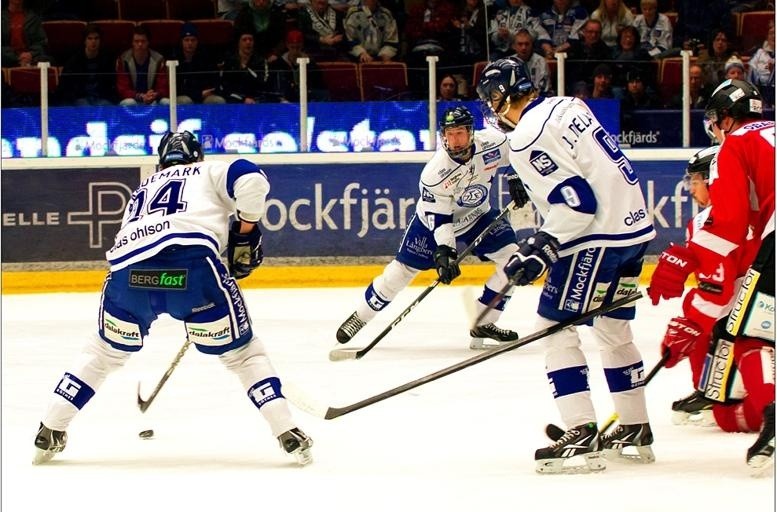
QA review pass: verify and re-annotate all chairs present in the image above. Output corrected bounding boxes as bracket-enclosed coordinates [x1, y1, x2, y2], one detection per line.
[2, 0, 775, 103]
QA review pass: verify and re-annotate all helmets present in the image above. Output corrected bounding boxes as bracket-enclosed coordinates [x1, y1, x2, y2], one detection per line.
[476, 56, 534, 134]
[683, 145, 721, 192]
[158, 130, 202, 168]
[439, 106, 474, 158]
[703, 79, 764, 143]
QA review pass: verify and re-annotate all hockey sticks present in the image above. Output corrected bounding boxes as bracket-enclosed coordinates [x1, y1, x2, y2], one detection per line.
[330, 201, 516, 360]
[547, 348, 671, 443]
[138, 251, 251, 412]
[302, 289, 655, 418]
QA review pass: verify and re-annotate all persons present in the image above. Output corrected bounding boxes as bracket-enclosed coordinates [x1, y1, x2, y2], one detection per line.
[26, 130, 315, 456]
[4, 0, 774, 122]
[331, 105, 528, 344]
[641, 79, 775, 472]
[473, 57, 657, 462]
[642, 142, 765, 433]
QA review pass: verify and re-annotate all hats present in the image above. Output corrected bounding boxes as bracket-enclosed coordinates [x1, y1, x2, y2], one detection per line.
[724, 55, 744, 75]
[180, 22, 197, 42]
[288, 30, 303, 45]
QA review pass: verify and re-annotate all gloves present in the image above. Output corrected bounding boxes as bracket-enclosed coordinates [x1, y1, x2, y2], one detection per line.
[507, 172, 529, 208]
[504, 232, 560, 286]
[433, 245, 461, 284]
[228, 221, 264, 279]
[649, 243, 698, 305]
[661, 316, 704, 368]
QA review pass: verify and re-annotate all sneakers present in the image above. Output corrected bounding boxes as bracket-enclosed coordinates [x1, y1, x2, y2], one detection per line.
[672, 389, 715, 414]
[470, 322, 518, 342]
[276, 427, 313, 454]
[34, 422, 67, 452]
[534, 423, 653, 461]
[337, 311, 366, 344]
